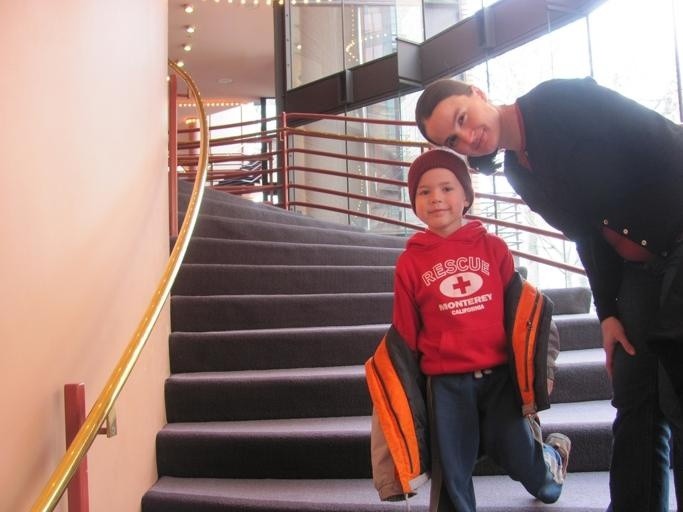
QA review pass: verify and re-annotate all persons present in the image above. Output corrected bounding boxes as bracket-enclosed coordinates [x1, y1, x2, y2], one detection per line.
[361, 147, 572, 512]
[415, 76, 681, 511]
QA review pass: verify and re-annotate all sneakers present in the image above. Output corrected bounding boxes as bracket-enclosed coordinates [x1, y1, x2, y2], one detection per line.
[540, 434, 572, 502]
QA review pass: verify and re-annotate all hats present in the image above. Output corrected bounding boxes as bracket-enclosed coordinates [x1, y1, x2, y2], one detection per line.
[408, 151, 474, 217]
